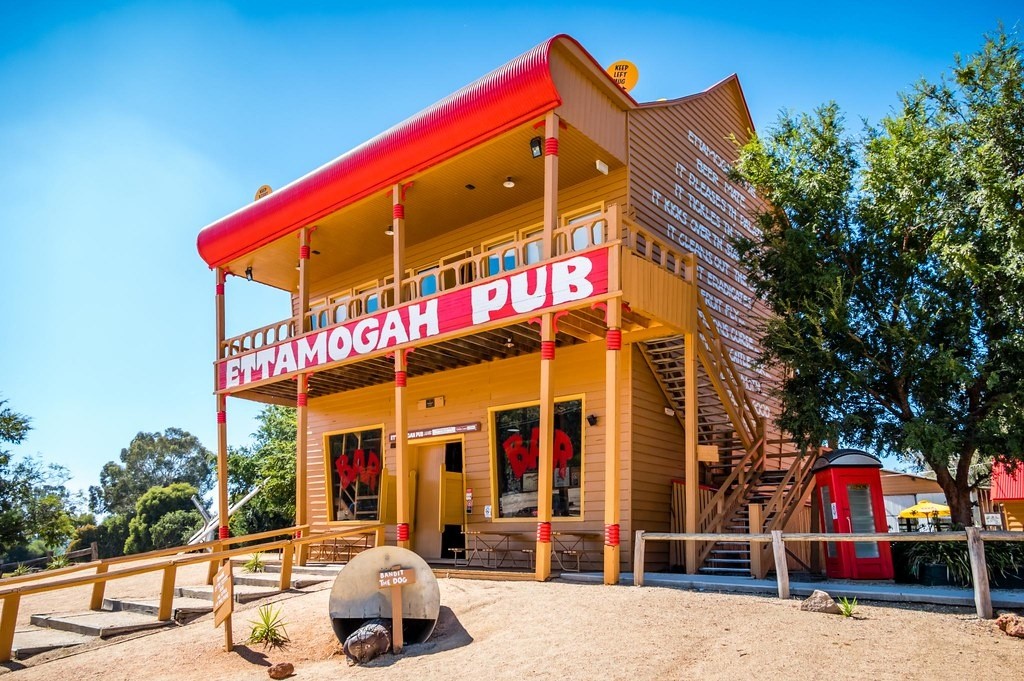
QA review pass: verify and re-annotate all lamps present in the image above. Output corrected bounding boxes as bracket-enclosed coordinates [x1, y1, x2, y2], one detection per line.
[295, 260, 300, 271]
[308, 384, 313, 392]
[384, 226, 394, 236]
[245, 267, 253, 282]
[503, 337, 515, 349]
[503, 176, 515, 188]
[530, 137, 543, 158]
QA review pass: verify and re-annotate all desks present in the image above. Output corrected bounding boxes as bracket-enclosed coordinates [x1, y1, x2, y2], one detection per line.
[288, 532, 325, 561]
[550, 531, 600, 572]
[461, 530, 523, 569]
[326, 529, 375, 562]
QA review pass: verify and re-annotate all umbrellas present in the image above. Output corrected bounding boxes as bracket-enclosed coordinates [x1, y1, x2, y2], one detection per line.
[897, 500, 952, 532]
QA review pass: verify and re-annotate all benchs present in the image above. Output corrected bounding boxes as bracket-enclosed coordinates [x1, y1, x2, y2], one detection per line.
[522, 549, 567, 571]
[449, 548, 490, 567]
[564, 550, 604, 573]
[279, 543, 373, 562]
[482, 548, 530, 571]
